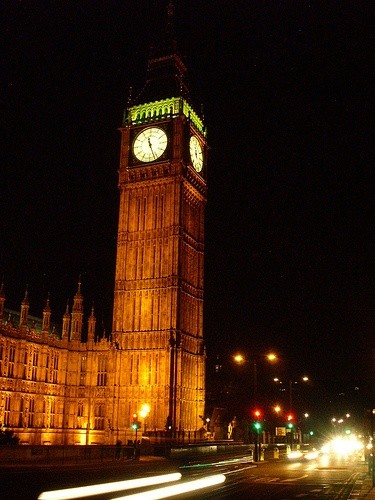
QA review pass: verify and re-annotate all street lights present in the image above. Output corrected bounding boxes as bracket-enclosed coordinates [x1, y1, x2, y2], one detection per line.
[272, 375, 309, 443]
[232, 353, 277, 461]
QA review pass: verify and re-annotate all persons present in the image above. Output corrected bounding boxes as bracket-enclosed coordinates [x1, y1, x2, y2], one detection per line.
[127, 440, 133, 459]
[135, 440, 141, 460]
[115, 440, 122, 461]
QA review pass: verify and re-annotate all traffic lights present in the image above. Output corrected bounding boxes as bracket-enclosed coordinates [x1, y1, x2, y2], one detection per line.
[133, 420, 141, 429]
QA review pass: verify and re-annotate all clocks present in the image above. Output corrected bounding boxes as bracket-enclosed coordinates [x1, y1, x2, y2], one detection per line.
[189, 135, 204, 172]
[132, 126, 168, 163]
